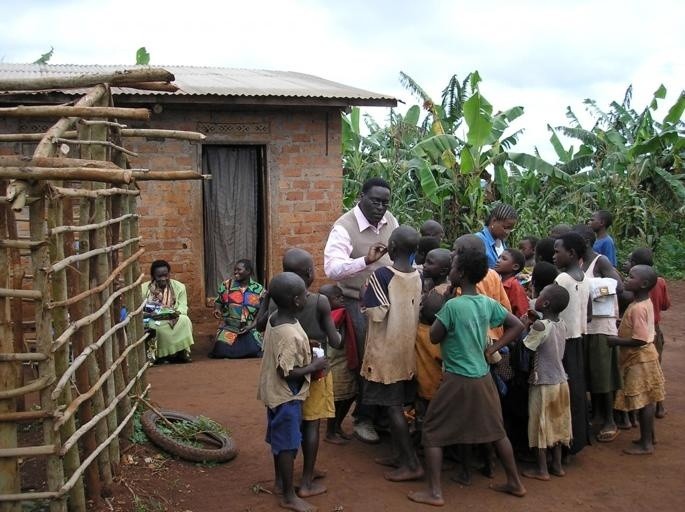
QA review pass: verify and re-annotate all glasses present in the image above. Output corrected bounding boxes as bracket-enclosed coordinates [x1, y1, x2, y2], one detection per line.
[364, 196, 390, 207]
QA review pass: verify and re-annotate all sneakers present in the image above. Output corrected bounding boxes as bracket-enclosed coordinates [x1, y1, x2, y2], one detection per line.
[352, 419, 380, 444]
[182, 352, 193, 363]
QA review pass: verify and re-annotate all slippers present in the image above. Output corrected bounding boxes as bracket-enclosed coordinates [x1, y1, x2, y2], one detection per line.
[597, 430, 621, 442]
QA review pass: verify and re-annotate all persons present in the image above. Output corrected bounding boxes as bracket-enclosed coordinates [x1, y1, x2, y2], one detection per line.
[257, 249, 344, 498]
[254, 271, 328, 511]
[209, 258, 268, 356]
[141, 260, 195, 364]
[321, 179, 399, 440]
[320, 200, 670, 506]
